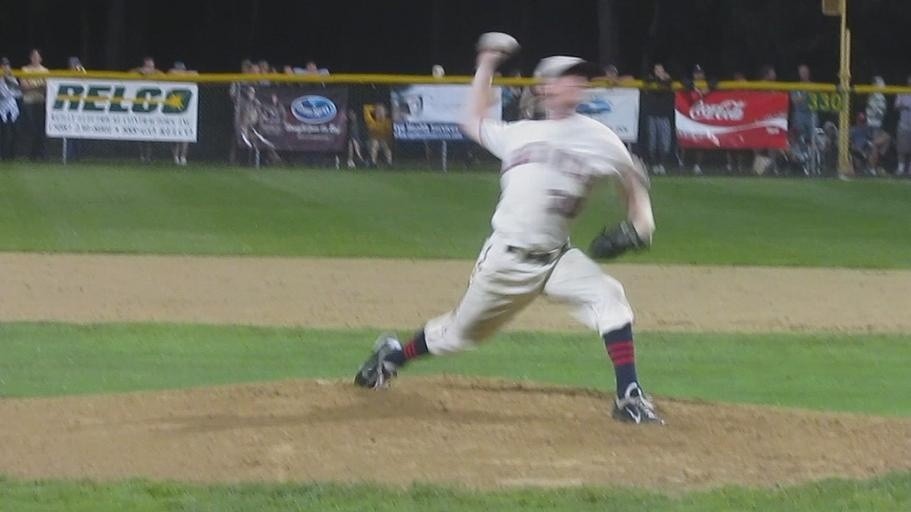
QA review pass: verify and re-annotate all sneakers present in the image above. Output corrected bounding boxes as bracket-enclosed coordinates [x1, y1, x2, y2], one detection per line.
[612, 381, 665, 425]
[355, 336, 402, 389]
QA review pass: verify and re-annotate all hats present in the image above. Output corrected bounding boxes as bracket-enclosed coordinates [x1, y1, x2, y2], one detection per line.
[532, 57, 586, 84]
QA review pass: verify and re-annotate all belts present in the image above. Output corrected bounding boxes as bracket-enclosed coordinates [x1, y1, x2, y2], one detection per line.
[509, 242, 567, 260]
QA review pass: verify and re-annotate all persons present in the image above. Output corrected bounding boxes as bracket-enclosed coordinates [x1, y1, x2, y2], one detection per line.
[230, 61, 393, 169]
[591, 64, 911, 177]
[0, 48, 198, 165]
[352, 26, 664, 431]
[461, 72, 545, 169]
[432, 64, 445, 77]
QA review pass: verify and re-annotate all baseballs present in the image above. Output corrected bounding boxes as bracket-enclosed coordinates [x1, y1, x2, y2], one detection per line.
[483, 33, 520, 56]
